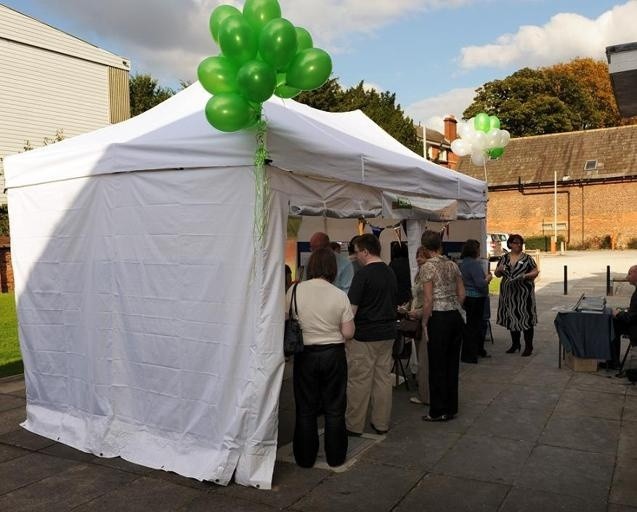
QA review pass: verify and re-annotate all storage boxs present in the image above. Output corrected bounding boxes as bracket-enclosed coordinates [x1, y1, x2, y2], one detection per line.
[564, 347, 599, 373]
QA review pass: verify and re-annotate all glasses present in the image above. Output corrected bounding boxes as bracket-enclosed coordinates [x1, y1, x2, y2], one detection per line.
[511, 241, 522, 245]
[354, 248, 365, 254]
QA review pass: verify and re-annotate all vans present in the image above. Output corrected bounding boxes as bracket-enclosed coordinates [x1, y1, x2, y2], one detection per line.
[487, 232, 502, 261]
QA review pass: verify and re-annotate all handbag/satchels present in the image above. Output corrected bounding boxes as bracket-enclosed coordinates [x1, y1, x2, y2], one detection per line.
[399, 315, 421, 339]
[284, 284, 302, 354]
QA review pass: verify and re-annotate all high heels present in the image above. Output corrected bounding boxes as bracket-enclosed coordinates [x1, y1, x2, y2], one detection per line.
[505, 343, 520, 354]
[410, 396, 422, 406]
[521, 345, 533, 357]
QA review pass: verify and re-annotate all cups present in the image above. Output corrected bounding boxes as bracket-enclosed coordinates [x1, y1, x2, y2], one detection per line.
[396, 309, 409, 320]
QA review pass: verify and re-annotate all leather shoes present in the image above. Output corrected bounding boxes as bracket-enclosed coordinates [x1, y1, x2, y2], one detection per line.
[422, 415, 448, 422]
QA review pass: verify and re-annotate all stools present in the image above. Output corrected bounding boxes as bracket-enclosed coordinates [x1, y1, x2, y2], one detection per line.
[616, 333, 636, 376]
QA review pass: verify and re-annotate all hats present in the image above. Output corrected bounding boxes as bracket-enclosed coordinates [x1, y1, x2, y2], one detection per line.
[310, 232, 329, 247]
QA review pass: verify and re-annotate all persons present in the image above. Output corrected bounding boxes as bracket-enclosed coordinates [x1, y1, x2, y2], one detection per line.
[598, 265, 637, 370]
[285, 247, 358, 470]
[345, 232, 399, 437]
[495, 234, 540, 357]
[286, 229, 491, 425]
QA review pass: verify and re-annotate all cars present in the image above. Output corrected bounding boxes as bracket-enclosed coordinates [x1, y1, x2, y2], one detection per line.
[498, 232, 526, 258]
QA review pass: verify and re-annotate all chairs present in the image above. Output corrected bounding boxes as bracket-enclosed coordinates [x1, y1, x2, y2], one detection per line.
[387, 323, 419, 392]
[482, 298, 494, 345]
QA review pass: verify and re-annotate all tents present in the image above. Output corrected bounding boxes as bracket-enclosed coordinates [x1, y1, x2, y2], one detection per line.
[1, 76, 489, 492]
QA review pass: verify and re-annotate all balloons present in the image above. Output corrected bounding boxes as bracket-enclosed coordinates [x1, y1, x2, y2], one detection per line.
[451, 113, 510, 166]
[197, 1, 333, 133]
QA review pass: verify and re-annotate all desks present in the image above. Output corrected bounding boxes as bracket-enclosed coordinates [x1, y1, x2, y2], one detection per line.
[555, 309, 623, 373]
